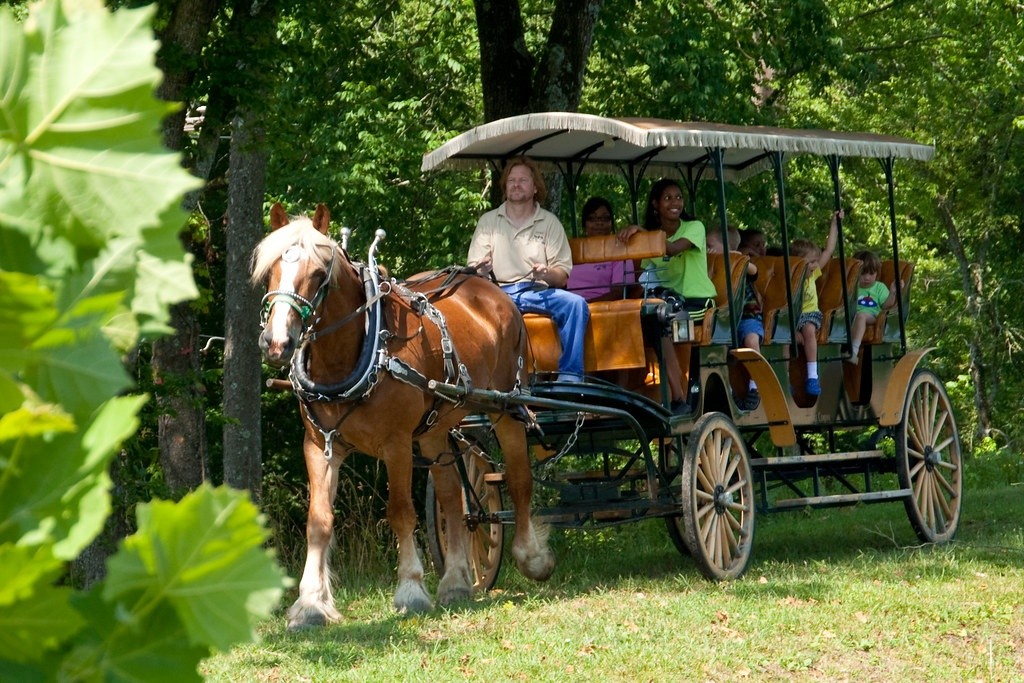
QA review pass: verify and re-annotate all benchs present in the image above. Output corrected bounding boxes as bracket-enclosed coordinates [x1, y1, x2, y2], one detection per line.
[522, 228, 666, 407]
[731, 255, 812, 403]
[636, 250, 749, 402]
[791, 257, 865, 403]
[845, 258, 918, 402]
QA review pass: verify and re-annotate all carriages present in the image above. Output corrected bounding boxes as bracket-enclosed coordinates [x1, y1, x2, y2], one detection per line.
[246, 111, 965, 630]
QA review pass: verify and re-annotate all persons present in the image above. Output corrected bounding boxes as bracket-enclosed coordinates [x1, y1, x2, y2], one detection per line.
[706, 223, 764, 409]
[468, 156, 587, 384]
[738, 228, 765, 254]
[789, 209, 844, 396]
[848, 250, 904, 365]
[568, 196, 634, 303]
[615, 177, 716, 409]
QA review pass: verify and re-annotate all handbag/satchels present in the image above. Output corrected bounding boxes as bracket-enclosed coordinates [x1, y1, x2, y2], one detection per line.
[637, 284, 686, 316]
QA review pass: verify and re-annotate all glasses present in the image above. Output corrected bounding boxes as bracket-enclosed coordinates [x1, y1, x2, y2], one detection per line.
[586, 212, 612, 222]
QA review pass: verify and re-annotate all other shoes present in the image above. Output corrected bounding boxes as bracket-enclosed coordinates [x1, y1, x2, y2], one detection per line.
[670, 399, 693, 415]
[847, 351, 858, 366]
[807, 374, 821, 395]
[557, 374, 582, 382]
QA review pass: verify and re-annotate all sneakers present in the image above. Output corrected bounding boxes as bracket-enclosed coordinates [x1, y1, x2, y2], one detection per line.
[745, 387, 760, 409]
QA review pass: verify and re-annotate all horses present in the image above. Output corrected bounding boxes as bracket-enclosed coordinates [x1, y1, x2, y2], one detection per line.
[252, 202, 556, 626]
